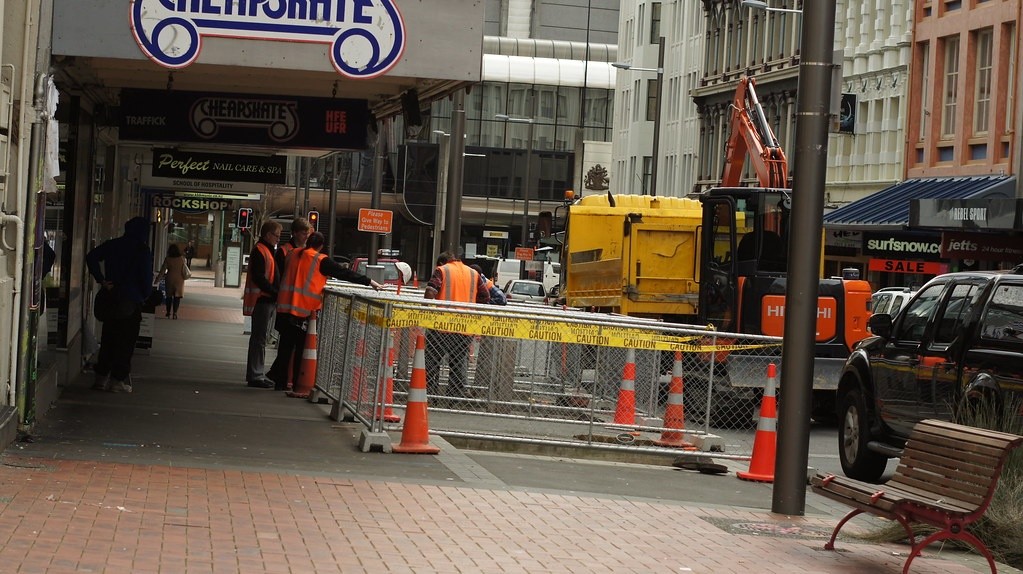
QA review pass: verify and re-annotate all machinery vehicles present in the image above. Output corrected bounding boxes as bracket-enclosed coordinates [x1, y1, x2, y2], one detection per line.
[679, 73, 875, 427]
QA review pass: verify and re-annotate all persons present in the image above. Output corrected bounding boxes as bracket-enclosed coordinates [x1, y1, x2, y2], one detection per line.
[87, 216, 155, 392]
[469, 263, 507, 387]
[264, 216, 313, 382]
[184, 241, 194, 271]
[275, 232, 389, 395]
[242, 220, 284, 388]
[154, 244, 186, 321]
[421, 250, 489, 411]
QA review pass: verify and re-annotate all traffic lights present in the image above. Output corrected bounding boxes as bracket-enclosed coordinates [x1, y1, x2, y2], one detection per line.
[238, 208, 248, 228]
[307, 210, 319, 234]
[248, 212, 254, 225]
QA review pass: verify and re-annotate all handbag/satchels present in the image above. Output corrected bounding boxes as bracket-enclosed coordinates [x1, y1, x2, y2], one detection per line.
[181, 255, 192, 280]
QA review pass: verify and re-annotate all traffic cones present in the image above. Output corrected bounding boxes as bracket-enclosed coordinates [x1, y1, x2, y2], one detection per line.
[651, 351, 695, 447]
[344, 317, 372, 405]
[607, 347, 640, 426]
[286, 309, 326, 399]
[392, 333, 441, 455]
[359, 330, 401, 422]
[734, 363, 777, 483]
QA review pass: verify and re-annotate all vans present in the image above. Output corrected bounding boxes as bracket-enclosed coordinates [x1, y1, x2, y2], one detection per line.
[349, 257, 404, 287]
[471, 254, 562, 297]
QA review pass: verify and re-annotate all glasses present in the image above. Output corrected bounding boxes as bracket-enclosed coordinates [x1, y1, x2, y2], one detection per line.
[272, 233, 283, 239]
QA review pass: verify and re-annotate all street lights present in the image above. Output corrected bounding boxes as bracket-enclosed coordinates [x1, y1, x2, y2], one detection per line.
[612, 63, 664, 196]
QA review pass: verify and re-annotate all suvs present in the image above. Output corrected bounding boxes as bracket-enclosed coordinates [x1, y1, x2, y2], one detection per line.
[836, 264, 1023, 481]
[870, 286, 919, 324]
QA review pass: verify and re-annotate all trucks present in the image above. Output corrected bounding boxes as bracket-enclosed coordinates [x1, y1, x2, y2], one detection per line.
[537, 189, 826, 317]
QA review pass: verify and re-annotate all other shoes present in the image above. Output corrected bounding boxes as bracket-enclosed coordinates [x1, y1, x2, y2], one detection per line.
[93, 376, 110, 389]
[248, 378, 274, 387]
[109, 378, 133, 393]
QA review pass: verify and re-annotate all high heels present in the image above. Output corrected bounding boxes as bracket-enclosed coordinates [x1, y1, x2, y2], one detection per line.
[166, 312, 171, 319]
[173, 312, 177, 320]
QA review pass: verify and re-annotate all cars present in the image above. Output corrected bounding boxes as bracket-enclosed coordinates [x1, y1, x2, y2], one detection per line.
[500, 279, 550, 307]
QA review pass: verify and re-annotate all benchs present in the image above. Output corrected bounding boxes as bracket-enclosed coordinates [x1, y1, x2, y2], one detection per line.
[811, 418, 1023, 574]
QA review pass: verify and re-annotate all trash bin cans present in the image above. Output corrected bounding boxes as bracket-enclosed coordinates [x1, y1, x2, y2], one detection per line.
[215, 261, 224, 288]
